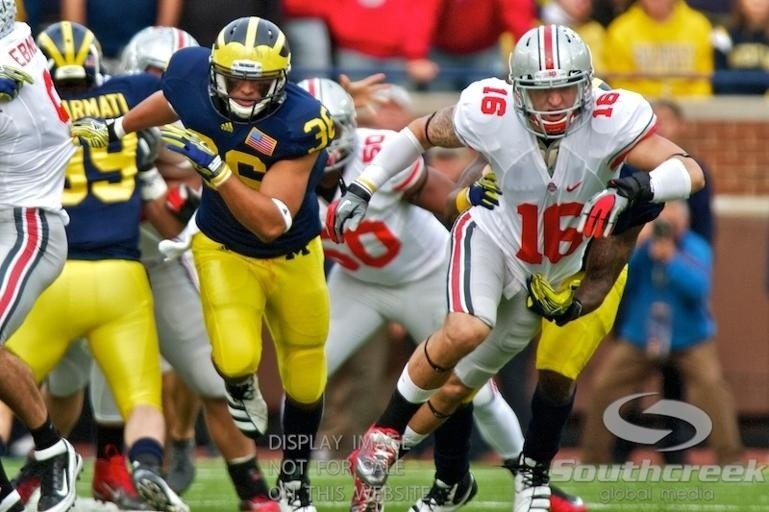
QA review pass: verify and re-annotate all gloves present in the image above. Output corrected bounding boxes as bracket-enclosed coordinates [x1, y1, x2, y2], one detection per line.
[457, 172, 503, 212]
[327, 178, 374, 244]
[160, 124, 230, 191]
[525, 272, 581, 327]
[577, 187, 635, 240]
[69, 116, 119, 149]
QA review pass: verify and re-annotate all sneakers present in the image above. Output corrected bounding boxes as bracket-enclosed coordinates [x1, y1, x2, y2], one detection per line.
[355, 423, 401, 487]
[93, 454, 195, 512]
[346, 447, 384, 512]
[224, 377, 270, 439]
[240, 475, 317, 511]
[409, 470, 477, 512]
[0, 438, 84, 512]
[502, 454, 584, 512]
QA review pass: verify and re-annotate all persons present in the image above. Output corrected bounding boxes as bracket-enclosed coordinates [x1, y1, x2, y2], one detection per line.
[1, 0, 768, 512]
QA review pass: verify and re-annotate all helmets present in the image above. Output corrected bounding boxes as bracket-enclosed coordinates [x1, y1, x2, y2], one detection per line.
[508, 25, 595, 140]
[207, 15, 291, 129]
[0, 0, 16, 39]
[120, 24, 201, 76]
[297, 78, 359, 175]
[35, 21, 101, 87]
